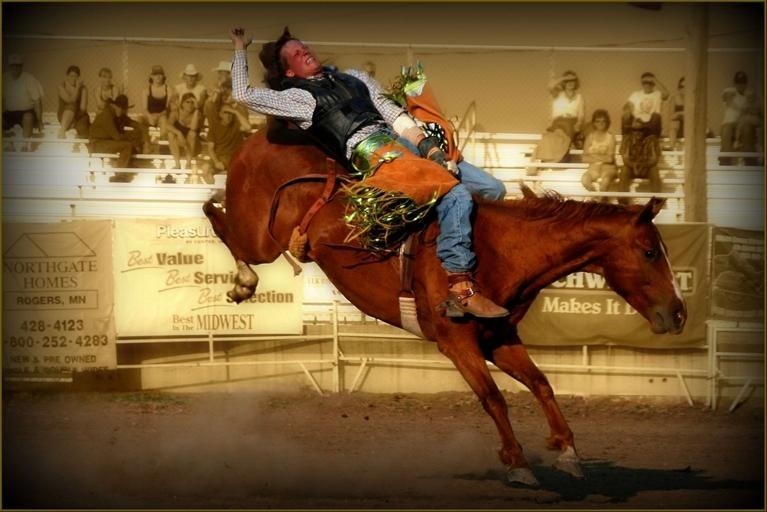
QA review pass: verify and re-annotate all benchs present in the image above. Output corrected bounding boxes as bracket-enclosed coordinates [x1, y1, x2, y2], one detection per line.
[0, 113, 259, 222]
[453, 129, 765, 231]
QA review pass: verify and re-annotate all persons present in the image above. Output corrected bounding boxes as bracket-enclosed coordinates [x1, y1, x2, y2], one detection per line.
[718, 72, 760, 166]
[2, 54, 45, 152]
[228, 25, 508, 319]
[88, 61, 254, 185]
[733, 86, 762, 149]
[57, 66, 91, 149]
[530, 71, 684, 207]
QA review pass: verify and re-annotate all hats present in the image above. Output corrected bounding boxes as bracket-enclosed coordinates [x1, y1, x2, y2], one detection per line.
[181, 64, 200, 77]
[258, 24, 290, 79]
[623, 117, 651, 130]
[8, 52, 25, 68]
[149, 64, 165, 76]
[211, 61, 233, 72]
[106, 92, 135, 110]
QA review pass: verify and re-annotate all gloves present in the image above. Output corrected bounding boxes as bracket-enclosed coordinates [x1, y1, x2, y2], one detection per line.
[417, 134, 449, 171]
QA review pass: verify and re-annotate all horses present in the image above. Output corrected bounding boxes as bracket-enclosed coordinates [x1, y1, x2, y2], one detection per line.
[200, 117, 689, 489]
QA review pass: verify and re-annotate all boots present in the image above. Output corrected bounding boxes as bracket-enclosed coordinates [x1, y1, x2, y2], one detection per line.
[437, 262, 511, 321]
